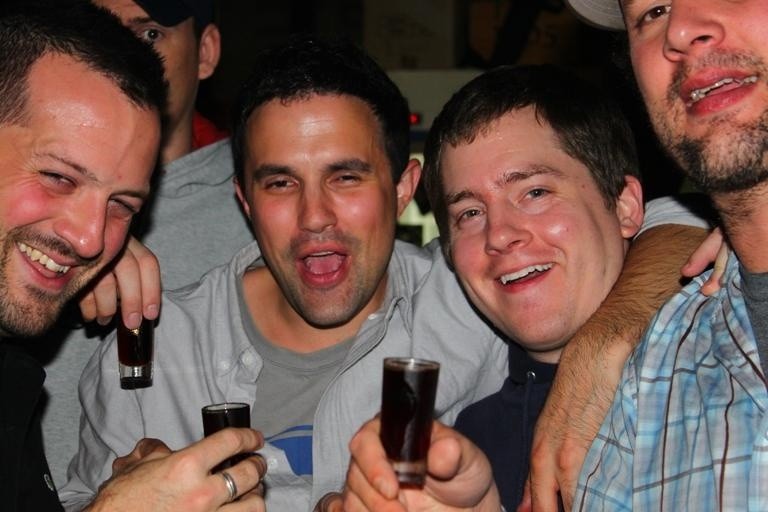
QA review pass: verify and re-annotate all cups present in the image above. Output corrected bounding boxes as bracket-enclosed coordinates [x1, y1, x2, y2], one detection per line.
[201, 399, 251, 475]
[381, 354, 441, 486]
[117, 307, 156, 388]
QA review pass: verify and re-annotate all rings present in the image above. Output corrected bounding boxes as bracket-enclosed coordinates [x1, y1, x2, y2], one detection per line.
[221, 469, 237, 503]
[318, 492, 339, 508]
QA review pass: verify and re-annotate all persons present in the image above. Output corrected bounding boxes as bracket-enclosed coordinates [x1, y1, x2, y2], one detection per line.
[0, 0, 768, 512]
